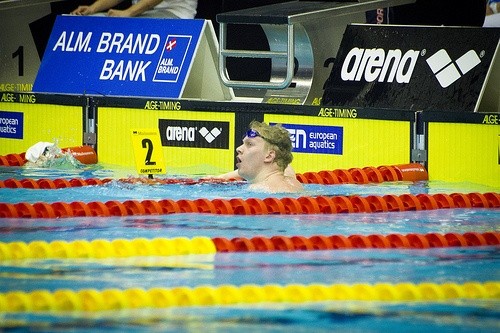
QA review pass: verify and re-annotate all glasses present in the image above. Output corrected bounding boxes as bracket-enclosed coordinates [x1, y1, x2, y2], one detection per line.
[240, 129, 274, 144]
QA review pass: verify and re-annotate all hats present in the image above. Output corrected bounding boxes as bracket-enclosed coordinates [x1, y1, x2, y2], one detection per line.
[25, 142, 54, 163]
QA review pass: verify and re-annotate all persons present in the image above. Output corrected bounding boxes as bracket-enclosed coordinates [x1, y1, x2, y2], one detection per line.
[235, 120, 305, 193]
[70, 0, 198, 19]
